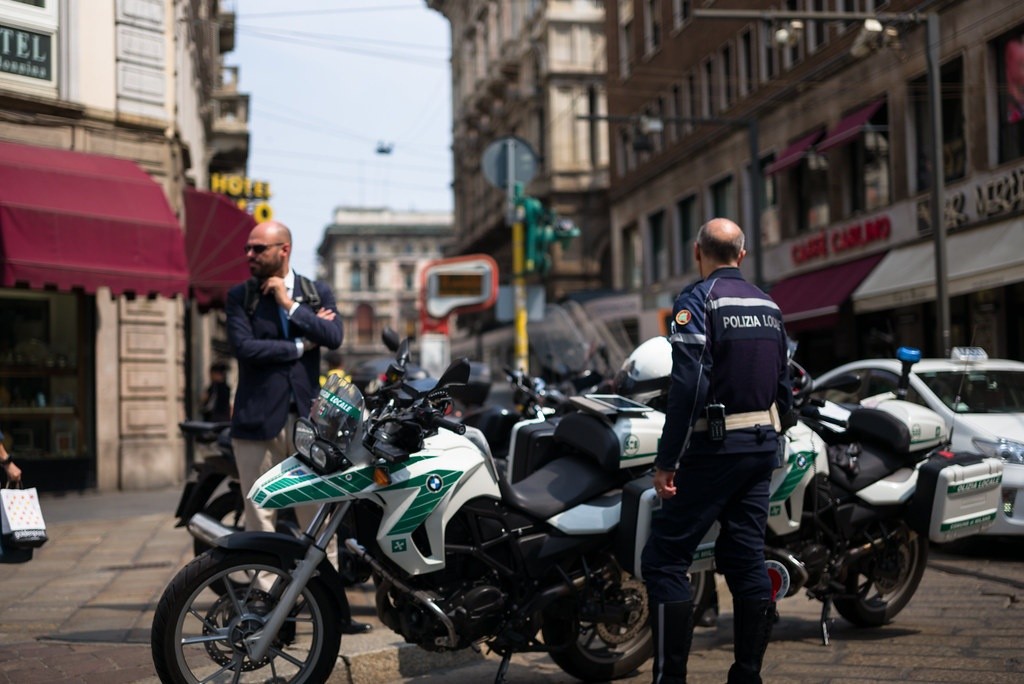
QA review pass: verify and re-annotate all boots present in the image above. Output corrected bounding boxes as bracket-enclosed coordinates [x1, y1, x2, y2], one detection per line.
[648, 600, 695, 684]
[727, 591, 775, 684]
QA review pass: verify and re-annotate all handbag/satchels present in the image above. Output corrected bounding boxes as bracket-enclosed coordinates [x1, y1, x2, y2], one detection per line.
[0, 478, 50, 564]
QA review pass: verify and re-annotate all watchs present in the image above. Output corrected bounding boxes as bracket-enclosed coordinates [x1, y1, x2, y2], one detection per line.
[0, 455, 13, 465]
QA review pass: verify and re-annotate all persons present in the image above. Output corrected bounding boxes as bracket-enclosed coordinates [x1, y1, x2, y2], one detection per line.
[0, 433, 22, 482]
[641, 219, 789, 684]
[222, 220, 374, 642]
[316, 347, 355, 388]
[195, 360, 233, 462]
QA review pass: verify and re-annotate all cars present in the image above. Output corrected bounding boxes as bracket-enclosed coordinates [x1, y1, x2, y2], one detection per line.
[347, 332, 625, 429]
[808, 347, 1024, 538]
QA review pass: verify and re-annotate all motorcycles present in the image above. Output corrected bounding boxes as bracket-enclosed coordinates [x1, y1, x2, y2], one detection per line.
[485, 363, 1010, 651]
[149, 332, 672, 683]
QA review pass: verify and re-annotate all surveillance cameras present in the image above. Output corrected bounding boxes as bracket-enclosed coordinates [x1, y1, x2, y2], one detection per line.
[878, 28, 898, 55]
[850, 18, 883, 58]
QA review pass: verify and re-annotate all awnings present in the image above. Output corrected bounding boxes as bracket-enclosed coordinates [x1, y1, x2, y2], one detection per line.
[763, 100, 891, 174]
[0, 137, 269, 318]
[767, 220, 1024, 335]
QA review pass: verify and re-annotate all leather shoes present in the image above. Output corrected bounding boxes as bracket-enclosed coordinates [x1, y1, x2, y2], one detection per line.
[342, 618, 373, 635]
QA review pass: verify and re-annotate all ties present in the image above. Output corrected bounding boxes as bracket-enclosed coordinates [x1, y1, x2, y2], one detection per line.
[277, 301, 289, 342]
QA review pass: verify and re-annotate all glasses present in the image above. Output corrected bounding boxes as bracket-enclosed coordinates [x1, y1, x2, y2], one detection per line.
[245, 243, 285, 254]
